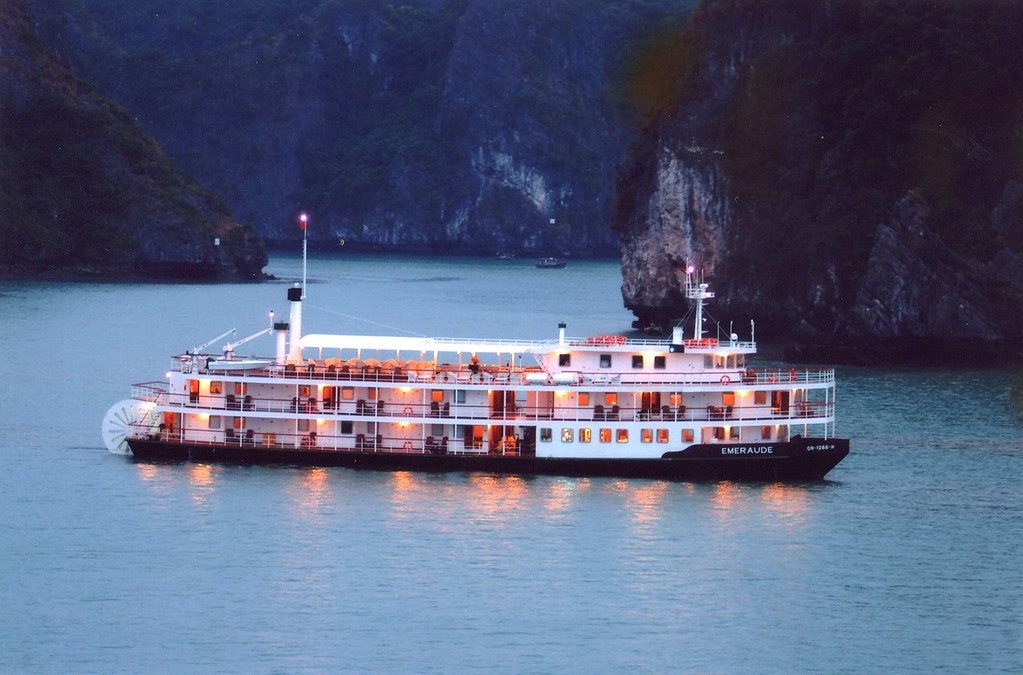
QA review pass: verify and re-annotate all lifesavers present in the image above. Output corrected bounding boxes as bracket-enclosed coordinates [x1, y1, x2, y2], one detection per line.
[403, 441, 413, 451]
[788, 370, 796, 380]
[768, 373, 779, 384]
[304, 436, 315, 448]
[402, 406, 414, 417]
[720, 375, 731, 386]
[305, 403, 316, 413]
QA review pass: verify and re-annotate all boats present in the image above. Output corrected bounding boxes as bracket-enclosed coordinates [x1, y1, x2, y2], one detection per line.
[534, 257, 567, 268]
[124, 211, 851, 484]
